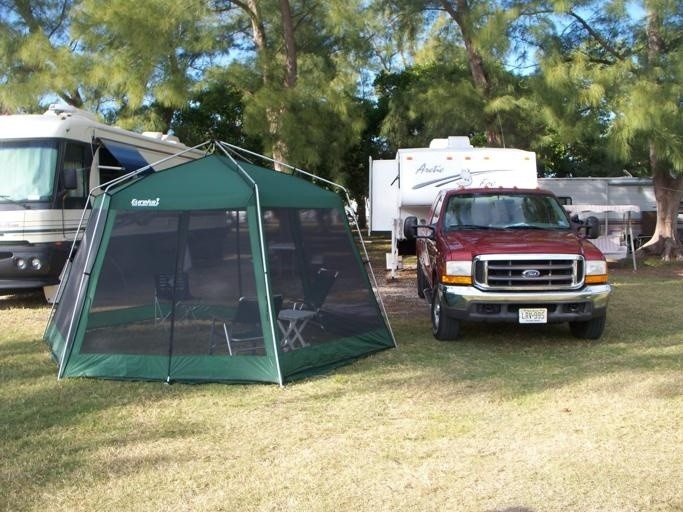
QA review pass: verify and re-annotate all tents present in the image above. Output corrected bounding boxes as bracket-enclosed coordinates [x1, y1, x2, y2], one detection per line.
[42, 134, 399, 387]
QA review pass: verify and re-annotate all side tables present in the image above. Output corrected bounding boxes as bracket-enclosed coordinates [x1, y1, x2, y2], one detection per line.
[276, 309, 317, 350]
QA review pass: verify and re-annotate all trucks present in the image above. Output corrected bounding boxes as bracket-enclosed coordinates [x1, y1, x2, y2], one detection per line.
[0, 105, 229, 297]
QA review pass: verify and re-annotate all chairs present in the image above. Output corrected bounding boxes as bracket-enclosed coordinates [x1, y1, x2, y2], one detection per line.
[208, 294, 283, 356]
[154, 271, 202, 334]
[283, 267, 339, 334]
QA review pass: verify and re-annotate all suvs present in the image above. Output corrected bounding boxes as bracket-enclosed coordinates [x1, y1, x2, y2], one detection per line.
[401, 183, 611, 342]
